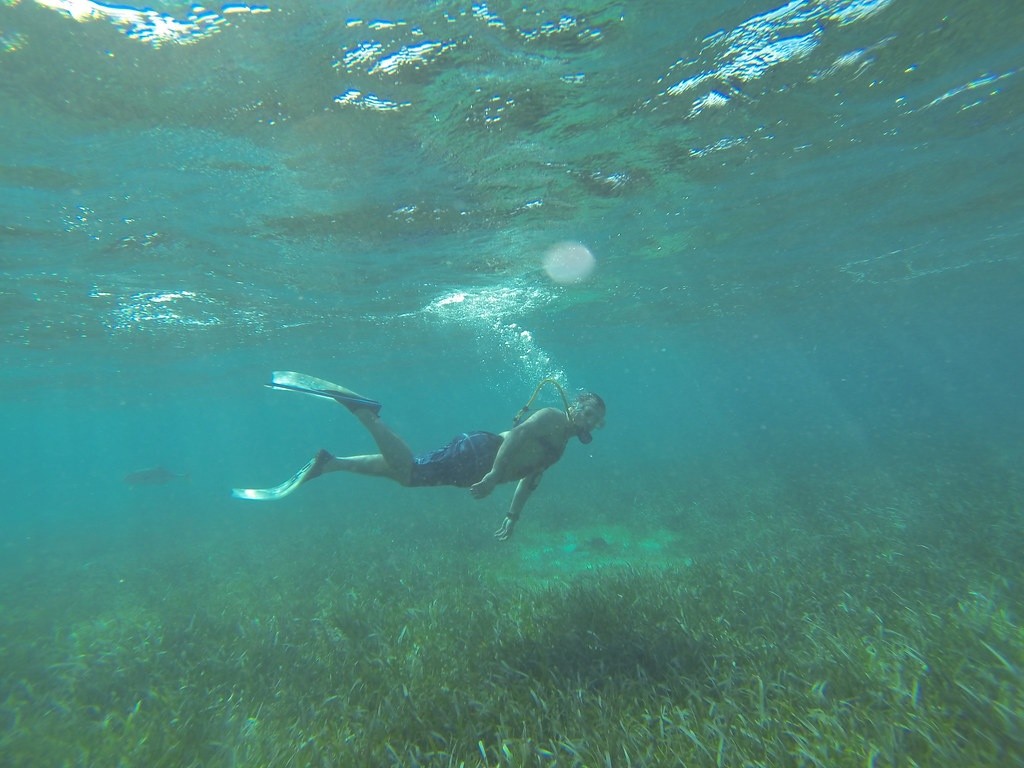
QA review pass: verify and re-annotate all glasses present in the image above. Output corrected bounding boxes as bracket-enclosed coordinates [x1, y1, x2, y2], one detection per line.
[583, 405, 605, 431]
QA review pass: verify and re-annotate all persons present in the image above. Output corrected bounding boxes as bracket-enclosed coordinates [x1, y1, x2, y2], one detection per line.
[227, 368, 607, 544]
